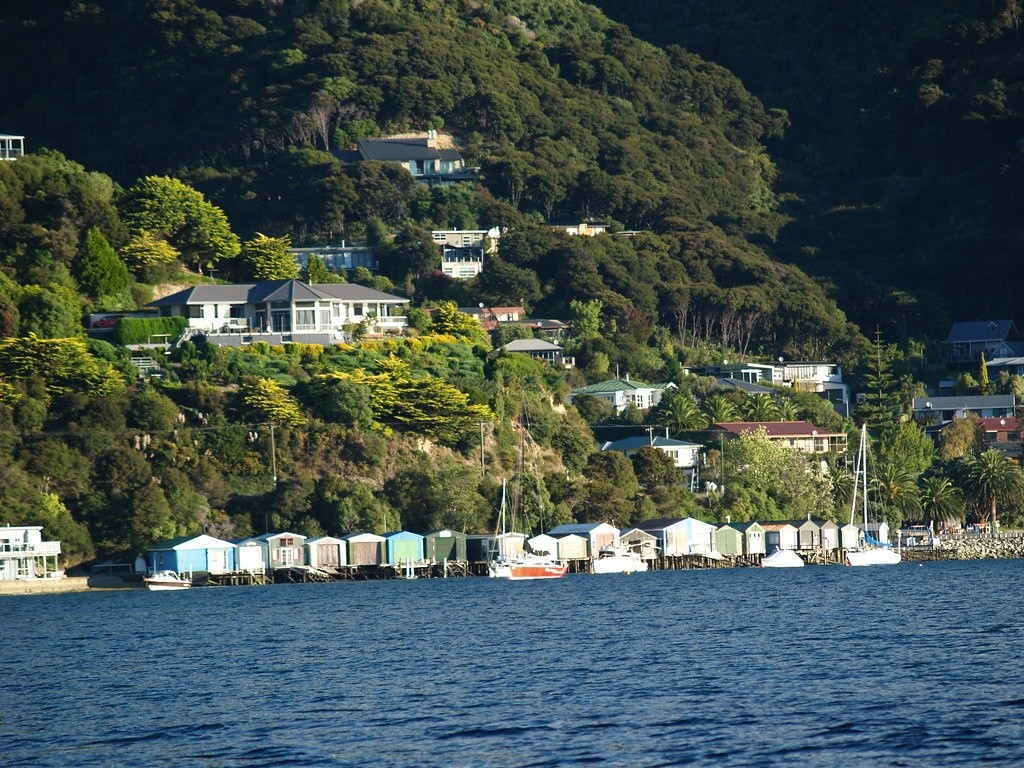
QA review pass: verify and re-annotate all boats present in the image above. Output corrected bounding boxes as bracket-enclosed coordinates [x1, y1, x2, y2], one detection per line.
[759, 546, 805, 570]
[143, 571, 193, 592]
[590, 547, 650, 575]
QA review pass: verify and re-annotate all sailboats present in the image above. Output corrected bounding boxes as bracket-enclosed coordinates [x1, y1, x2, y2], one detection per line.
[480, 477, 567, 581]
[842, 422, 902, 570]
